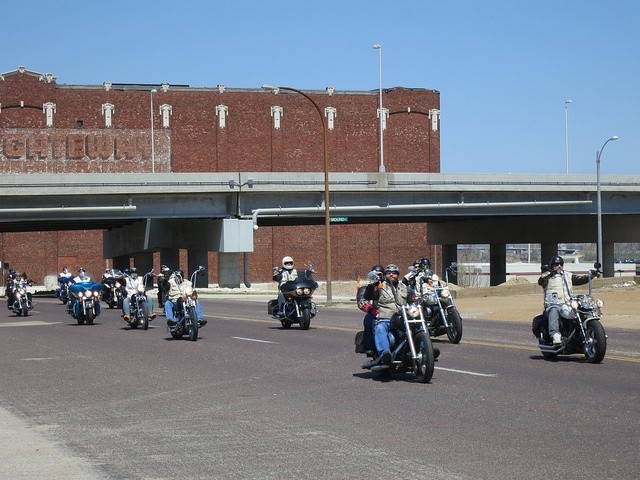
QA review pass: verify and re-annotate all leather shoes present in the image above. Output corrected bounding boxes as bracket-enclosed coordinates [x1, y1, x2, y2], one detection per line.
[366, 348, 374, 357]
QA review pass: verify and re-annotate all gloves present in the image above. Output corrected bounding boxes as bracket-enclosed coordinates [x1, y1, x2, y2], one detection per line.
[370, 307, 380, 316]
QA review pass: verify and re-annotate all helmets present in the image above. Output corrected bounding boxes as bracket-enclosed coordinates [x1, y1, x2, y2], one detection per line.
[130, 267, 138, 273]
[384, 264, 400, 274]
[176, 270, 184, 276]
[550, 256, 564, 264]
[412, 260, 421, 265]
[419, 258, 430, 269]
[281, 256, 294, 269]
[371, 265, 384, 272]
[10, 268, 16, 273]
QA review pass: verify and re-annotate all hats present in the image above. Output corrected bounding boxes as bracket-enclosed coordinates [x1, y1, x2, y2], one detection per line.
[161, 265, 170, 272]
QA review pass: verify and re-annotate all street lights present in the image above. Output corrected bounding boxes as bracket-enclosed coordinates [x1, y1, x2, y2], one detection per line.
[261, 84, 331, 303]
[565, 100, 573, 176]
[372, 44, 386, 172]
[596, 136, 618, 277]
[151, 89, 157, 175]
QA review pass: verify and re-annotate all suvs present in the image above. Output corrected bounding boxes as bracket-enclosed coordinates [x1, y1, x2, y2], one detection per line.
[558, 249, 583, 263]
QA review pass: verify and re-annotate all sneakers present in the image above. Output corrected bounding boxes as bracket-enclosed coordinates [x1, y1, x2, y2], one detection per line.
[380, 351, 392, 365]
[167, 320, 174, 326]
[148, 312, 157, 320]
[199, 319, 207, 325]
[553, 333, 562, 344]
[433, 348, 440, 360]
[124, 314, 130, 323]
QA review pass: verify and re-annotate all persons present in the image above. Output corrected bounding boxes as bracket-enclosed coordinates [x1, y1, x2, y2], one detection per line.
[402, 258, 449, 304]
[538, 258, 601, 346]
[272, 256, 299, 318]
[6, 269, 33, 309]
[57, 266, 94, 294]
[102, 263, 208, 328]
[357, 265, 415, 364]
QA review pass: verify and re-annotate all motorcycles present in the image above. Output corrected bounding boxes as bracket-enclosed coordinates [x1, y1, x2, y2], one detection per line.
[65, 282, 104, 325]
[533, 262, 606, 365]
[363, 269, 434, 383]
[118, 269, 153, 330]
[163, 265, 205, 341]
[102, 269, 128, 309]
[56, 275, 76, 304]
[268, 267, 318, 332]
[7, 275, 37, 316]
[404, 261, 462, 343]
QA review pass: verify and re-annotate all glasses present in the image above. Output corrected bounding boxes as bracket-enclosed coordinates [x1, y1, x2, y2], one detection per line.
[415, 266, 419, 269]
[555, 263, 563, 266]
